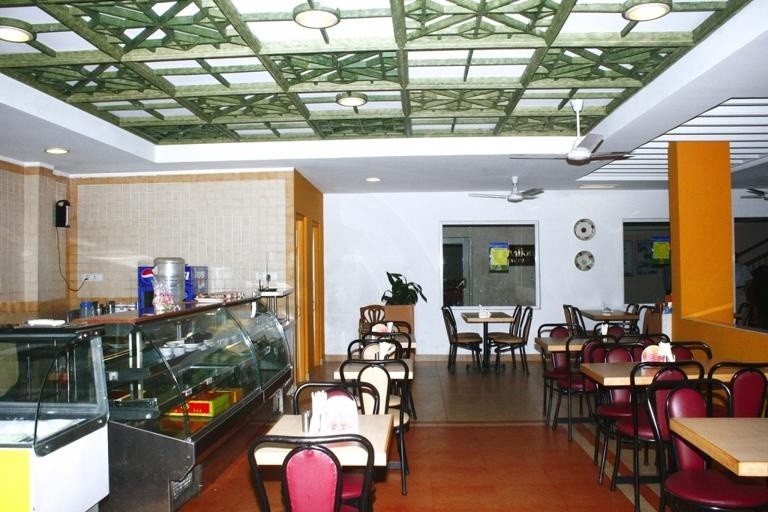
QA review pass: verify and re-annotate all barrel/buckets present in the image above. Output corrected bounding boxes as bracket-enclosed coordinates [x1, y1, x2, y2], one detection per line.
[153, 257, 186, 309]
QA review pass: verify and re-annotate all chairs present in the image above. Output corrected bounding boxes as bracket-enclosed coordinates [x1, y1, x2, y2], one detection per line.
[535, 322, 768, 491]
[247, 303, 417, 512]
[645, 378, 768, 511]
[440, 303, 534, 378]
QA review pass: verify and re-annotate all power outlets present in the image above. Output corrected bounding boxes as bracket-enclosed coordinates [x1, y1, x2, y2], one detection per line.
[82, 273, 104, 283]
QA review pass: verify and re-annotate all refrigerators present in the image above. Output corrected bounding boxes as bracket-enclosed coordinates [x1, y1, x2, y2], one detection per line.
[137, 265, 207, 314]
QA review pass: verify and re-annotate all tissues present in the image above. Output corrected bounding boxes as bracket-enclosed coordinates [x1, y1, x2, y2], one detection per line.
[322, 396, 360, 447]
[643, 342, 675, 369]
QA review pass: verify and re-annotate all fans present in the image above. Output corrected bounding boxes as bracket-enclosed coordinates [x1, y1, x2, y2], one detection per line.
[466, 174, 546, 203]
[506, 99, 642, 163]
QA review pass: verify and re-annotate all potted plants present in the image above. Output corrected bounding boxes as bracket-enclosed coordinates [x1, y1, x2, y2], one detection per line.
[380, 268, 428, 334]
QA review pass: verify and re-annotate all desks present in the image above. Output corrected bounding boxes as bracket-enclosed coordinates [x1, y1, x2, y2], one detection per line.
[669, 417, 768, 477]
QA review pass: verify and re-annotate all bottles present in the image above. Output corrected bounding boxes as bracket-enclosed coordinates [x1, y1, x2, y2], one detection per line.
[302, 408, 312, 433]
[80, 300, 116, 318]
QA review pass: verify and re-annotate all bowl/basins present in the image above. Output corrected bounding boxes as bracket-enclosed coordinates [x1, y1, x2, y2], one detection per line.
[158, 330, 238, 357]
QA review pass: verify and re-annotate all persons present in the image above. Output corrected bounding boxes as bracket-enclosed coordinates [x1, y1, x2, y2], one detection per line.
[736, 253, 753, 310]
